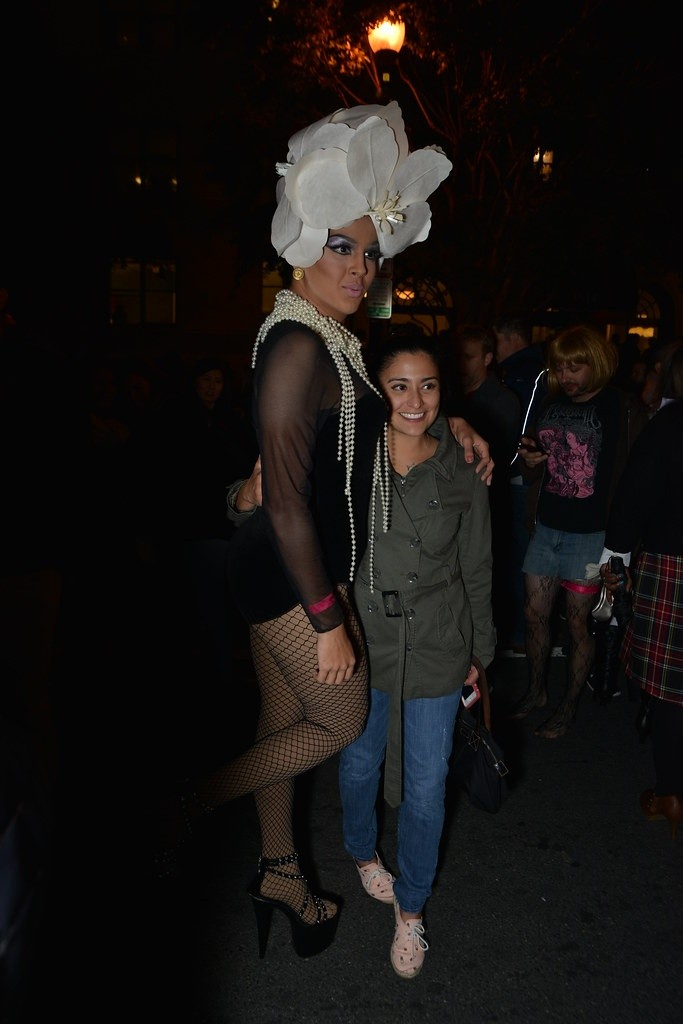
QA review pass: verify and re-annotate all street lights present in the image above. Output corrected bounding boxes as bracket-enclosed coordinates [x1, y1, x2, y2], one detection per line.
[365, 8, 410, 354]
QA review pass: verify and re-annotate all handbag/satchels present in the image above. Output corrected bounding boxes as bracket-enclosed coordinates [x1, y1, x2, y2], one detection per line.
[448, 655, 512, 814]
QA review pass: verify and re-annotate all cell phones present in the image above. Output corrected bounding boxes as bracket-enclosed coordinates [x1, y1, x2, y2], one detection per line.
[516, 441, 544, 456]
[460, 667, 482, 710]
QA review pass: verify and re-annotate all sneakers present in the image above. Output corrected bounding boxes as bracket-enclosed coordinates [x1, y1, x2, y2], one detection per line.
[354, 851, 398, 904]
[391, 902, 429, 979]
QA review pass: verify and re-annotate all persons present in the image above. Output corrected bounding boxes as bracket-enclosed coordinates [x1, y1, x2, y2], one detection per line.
[0, 285, 252, 1024]
[226, 324, 500, 976]
[446, 319, 683, 842]
[512, 323, 625, 739]
[145, 104, 493, 956]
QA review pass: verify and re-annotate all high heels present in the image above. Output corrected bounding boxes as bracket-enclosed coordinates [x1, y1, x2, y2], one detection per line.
[130, 788, 216, 901]
[639, 788, 683, 841]
[246, 851, 342, 959]
[635, 703, 655, 743]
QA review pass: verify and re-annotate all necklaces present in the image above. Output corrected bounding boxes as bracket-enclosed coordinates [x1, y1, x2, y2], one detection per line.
[251, 290, 391, 594]
